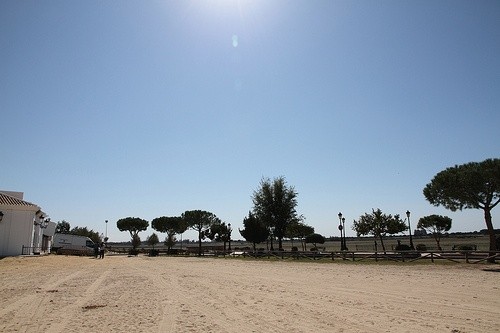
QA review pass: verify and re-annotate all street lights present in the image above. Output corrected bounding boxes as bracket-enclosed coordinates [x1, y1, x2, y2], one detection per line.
[338, 212, 347, 251]
[227, 223, 232, 250]
[105, 220, 108, 248]
[406, 210, 414, 249]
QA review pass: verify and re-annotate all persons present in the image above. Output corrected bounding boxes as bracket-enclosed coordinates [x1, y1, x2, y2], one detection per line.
[98, 242, 105, 259]
[94, 241, 98, 258]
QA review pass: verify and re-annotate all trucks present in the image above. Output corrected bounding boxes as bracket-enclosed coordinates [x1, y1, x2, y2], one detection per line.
[51, 232, 101, 255]
